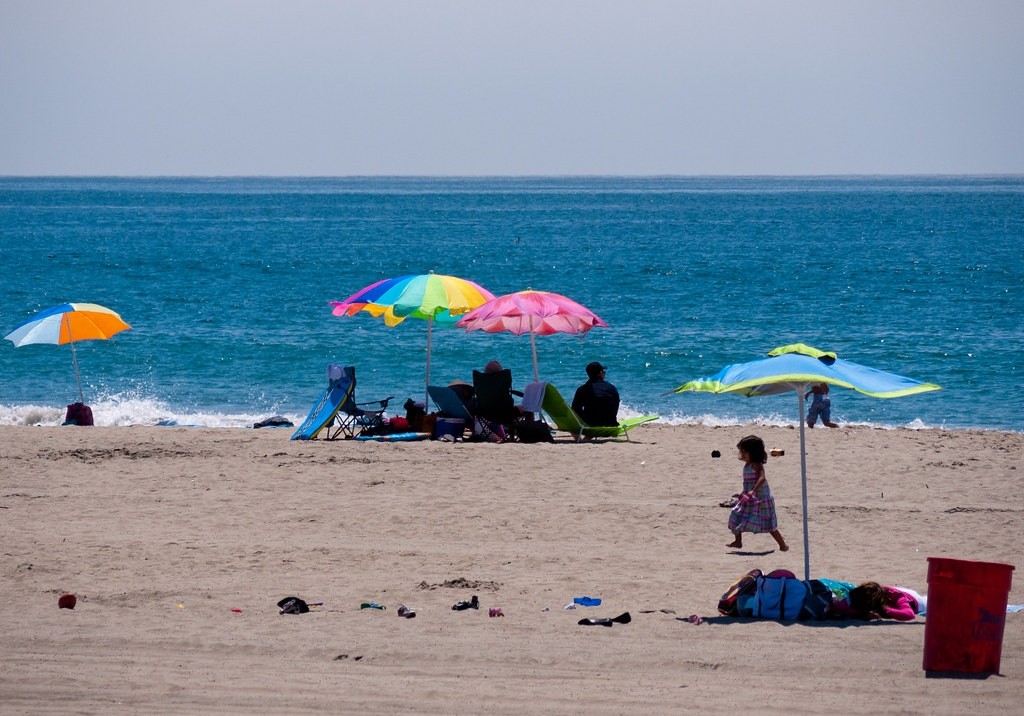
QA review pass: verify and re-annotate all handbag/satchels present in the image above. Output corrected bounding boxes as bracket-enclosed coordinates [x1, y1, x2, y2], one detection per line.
[718, 567, 855, 621]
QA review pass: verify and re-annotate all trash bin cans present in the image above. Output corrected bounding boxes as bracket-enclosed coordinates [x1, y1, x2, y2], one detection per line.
[922, 557, 1015, 676]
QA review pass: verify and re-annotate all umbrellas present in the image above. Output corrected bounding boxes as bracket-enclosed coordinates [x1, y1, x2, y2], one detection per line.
[453, 286, 607, 382]
[660, 341, 943, 580]
[3, 302, 133, 406]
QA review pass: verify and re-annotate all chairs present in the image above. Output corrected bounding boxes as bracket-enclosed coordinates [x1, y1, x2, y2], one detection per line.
[527, 380, 659, 442]
[472, 368, 523, 441]
[326, 365, 393, 440]
[427, 384, 481, 436]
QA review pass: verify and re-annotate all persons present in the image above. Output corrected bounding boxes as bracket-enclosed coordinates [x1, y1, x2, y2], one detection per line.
[485, 360, 535, 421]
[725, 436, 791, 552]
[804, 382, 839, 428]
[571, 362, 616, 440]
[833, 579, 927, 621]
[328, 270, 495, 414]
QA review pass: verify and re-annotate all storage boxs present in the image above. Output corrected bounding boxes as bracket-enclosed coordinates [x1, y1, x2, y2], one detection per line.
[435, 416, 466, 438]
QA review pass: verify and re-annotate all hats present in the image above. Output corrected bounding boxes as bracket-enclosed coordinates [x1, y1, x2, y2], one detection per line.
[585, 361, 608, 374]
